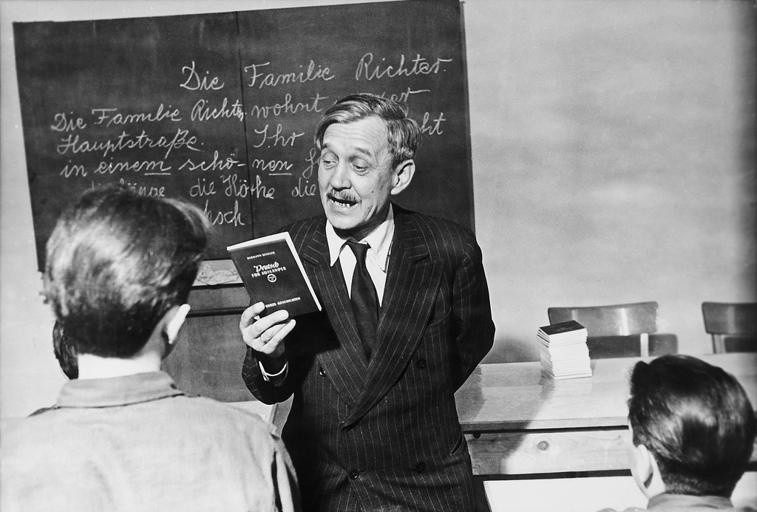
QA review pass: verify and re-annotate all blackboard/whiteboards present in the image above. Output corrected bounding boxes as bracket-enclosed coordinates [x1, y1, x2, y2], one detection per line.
[14, 0, 479, 282]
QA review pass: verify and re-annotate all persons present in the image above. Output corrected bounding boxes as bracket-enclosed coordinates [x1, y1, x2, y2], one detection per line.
[241, 93, 494, 512]
[624, 352, 756, 512]
[0, 184, 293, 512]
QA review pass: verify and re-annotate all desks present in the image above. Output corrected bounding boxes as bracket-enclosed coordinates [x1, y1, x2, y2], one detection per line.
[455, 354, 757, 476]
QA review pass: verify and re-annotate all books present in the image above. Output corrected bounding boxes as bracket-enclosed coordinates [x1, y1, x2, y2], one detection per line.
[536, 320, 591, 381]
[226, 231, 324, 320]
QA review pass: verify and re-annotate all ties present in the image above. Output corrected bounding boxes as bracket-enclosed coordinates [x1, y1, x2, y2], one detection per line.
[346, 240, 381, 362]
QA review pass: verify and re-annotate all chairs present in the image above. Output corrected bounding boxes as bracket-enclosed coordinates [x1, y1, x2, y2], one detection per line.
[549, 301, 660, 357]
[702, 302, 756, 354]
